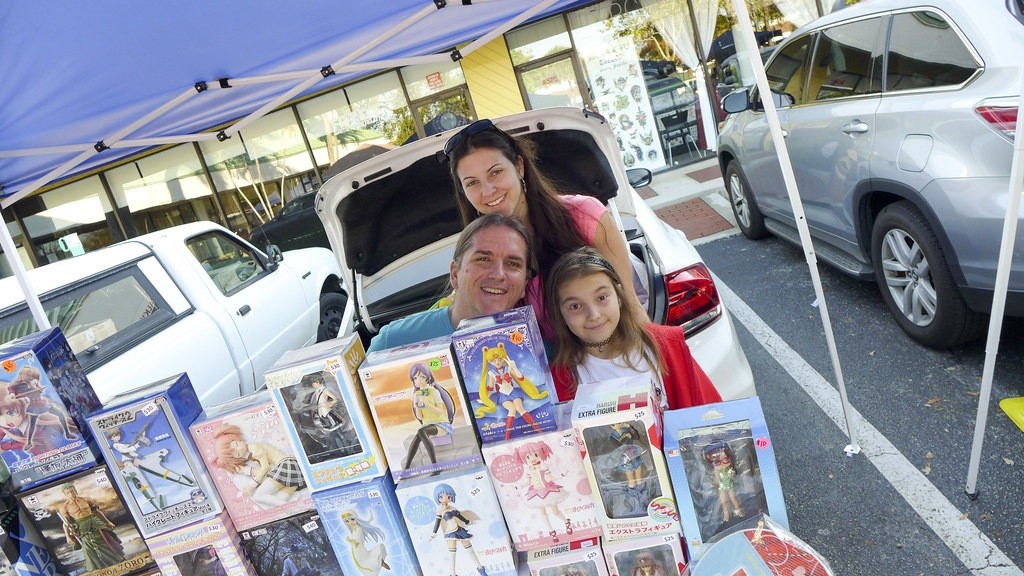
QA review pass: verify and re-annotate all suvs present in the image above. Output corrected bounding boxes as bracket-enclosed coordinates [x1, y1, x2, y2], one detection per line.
[315, 106, 758, 402]
[717, 0, 1024, 351]
[246, 189, 332, 251]
[719, 43, 779, 89]
[643, 77, 701, 149]
[2, 220, 358, 408]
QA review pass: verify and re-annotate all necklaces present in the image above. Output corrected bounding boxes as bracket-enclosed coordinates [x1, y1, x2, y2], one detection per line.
[582, 335, 615, 353]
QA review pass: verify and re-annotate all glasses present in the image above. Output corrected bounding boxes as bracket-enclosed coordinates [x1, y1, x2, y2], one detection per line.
[443, 119, 519, 163]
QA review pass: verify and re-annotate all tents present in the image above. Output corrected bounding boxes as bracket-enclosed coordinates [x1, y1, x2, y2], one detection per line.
[2, 0, 1024, 503]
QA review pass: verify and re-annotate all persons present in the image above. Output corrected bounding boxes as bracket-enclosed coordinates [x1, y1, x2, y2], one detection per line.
[443, 116, 655, 329]
[363, 212, 556, 372]
[561, 562, 586, 576]
[609, 420, 648, 500]
[308, 372, 354, 455]
[541, 244, 724, 412]
[704, 437, 748, 522]
[630, 551, 663, 576]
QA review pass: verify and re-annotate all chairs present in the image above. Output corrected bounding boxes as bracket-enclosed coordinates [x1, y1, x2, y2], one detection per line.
[660, 110, 704, 168]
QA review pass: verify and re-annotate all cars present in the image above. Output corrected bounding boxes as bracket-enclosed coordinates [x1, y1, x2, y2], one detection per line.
[588, 60, 676, 100]
[705, 27, 782, 63]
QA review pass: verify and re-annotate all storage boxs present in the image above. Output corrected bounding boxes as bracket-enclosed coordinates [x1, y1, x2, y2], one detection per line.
[0, 306, 793, 576]
